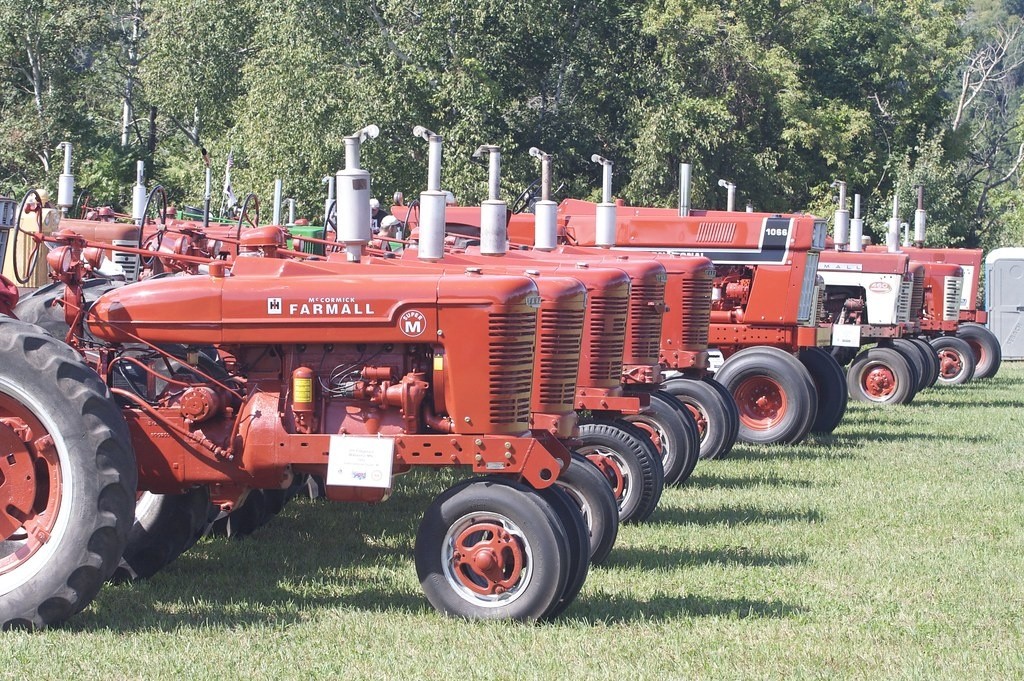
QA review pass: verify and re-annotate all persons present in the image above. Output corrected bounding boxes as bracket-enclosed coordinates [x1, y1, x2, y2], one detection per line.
[34, 188, 55, 208]
[368, 198, 400, 247]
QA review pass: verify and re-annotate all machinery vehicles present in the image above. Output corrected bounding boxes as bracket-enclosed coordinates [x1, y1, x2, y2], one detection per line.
[0, 125, 622, 637]
[0, 119, 1002, 527]
